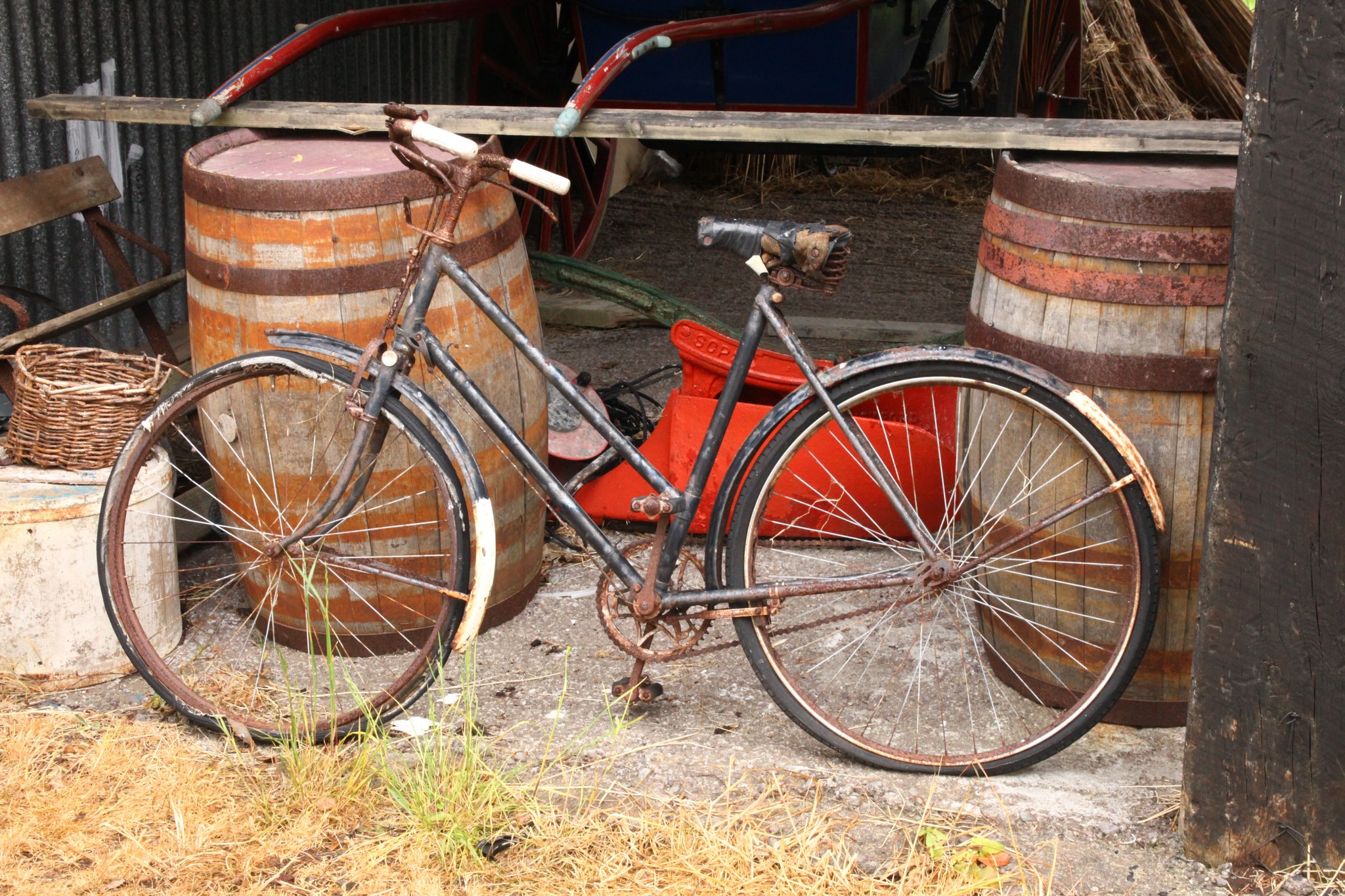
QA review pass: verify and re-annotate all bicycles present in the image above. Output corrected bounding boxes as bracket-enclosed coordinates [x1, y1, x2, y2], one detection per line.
[94, 100, 1171, 779]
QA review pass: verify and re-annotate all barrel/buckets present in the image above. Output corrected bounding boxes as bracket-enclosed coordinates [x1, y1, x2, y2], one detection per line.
[182, 126, 548, 658]
[956, 149, 1237, 728]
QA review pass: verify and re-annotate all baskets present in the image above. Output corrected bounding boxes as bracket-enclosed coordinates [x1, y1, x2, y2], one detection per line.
[10, 342, 170, 468]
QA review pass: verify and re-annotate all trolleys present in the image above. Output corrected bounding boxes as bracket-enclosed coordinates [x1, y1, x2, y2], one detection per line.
[190, 0, 1085, 292]
